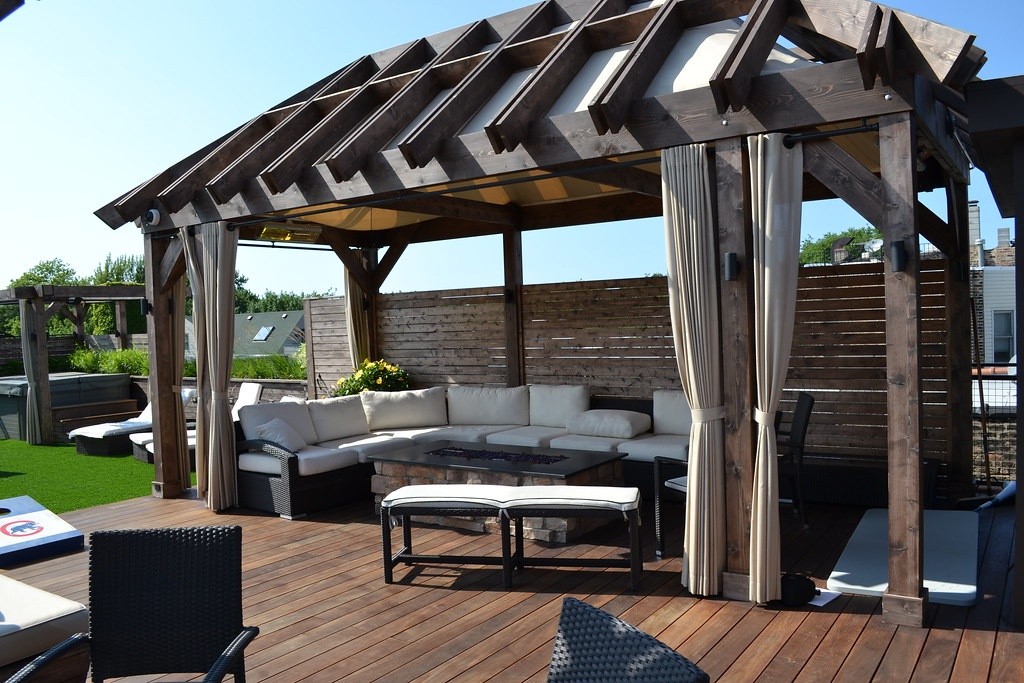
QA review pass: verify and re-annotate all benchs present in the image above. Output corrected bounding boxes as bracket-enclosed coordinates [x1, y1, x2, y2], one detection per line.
[379, 484, 642, 592]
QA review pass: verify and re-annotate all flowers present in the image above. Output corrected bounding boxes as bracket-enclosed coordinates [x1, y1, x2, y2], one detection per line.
[321, 356, 410, 399]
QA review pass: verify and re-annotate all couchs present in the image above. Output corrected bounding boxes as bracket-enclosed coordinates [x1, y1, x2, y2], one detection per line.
[232, 384, 691, 521]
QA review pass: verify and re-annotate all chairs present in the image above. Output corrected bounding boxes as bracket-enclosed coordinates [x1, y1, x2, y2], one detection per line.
[66, 387, 197, 454]
[650, 407, 784, 560]
[759, 389, 815, 537]
[127, 381, 264, 464]
[4, 525, 260, 683]
[545, 597, 711, 682]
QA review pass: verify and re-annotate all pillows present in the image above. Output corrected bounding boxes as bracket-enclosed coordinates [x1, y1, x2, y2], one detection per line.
[253, 417, 309, 454]
